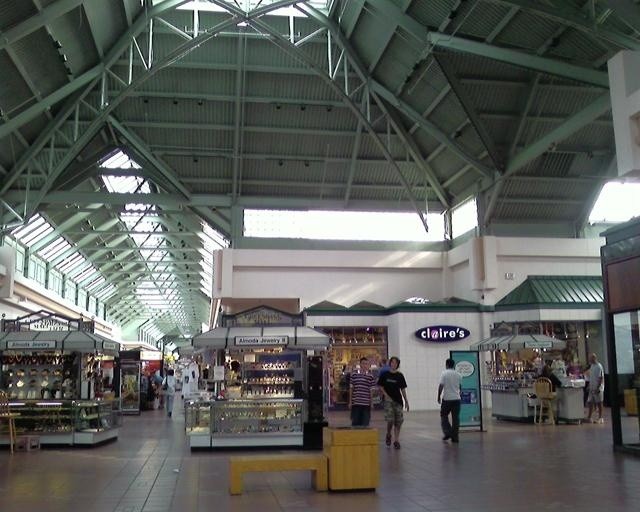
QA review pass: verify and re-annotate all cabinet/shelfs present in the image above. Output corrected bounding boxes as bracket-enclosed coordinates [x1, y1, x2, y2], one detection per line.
[0, 397, 123, 449]
[481, 379, 535, 423]
[555, 386, 584, 425]
[185, 399, 305, 448]
[242, 350, 307, 400]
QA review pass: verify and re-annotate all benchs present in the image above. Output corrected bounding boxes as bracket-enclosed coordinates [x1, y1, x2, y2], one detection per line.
[228, 454, 329, 496]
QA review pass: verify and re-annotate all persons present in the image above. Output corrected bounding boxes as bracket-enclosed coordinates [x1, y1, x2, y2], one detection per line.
[229, 361, 240, 384]
[539, 351, 605, 424]
[160, 370, 176, 417]
[347, 357, 376, 427]
[149, 371, 165, 410]
[376, 357, 409, 448]
[438, 358, 464, 441]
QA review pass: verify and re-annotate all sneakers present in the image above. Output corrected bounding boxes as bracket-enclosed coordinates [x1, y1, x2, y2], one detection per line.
[162, 383, 169, 390]
[594, 418, 603, 423]
[385, 433, 391, 446]
[442, 434, 453, 440]
[393, 440, 400, 449]
[585, 418, 593, 422]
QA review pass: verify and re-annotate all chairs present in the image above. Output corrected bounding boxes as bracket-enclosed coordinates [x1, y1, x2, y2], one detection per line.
[534, 376, 557, 425]
[0, 389, 22, 455]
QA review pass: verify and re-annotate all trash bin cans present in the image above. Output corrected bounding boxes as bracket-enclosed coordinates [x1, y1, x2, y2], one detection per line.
[624, 388, 639, 416]
[303, 421, 329, 451]
